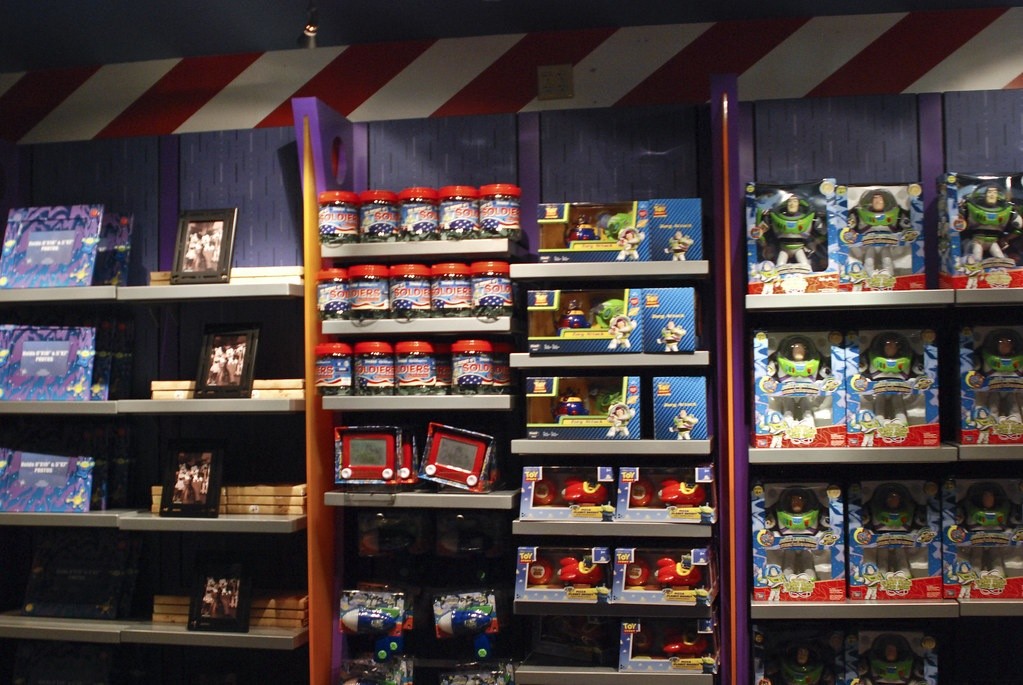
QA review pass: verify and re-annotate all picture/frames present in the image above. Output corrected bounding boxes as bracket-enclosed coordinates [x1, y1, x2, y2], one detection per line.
[194, 323, 262, 396]
[168, 206, 238, 282]
[157, 438, 223, 517]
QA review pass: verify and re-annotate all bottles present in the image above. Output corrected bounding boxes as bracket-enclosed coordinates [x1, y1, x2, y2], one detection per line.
[313, 185, 522, 395]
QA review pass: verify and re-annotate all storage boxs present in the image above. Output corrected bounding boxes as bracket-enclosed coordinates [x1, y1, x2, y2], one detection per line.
[612, 545, 717, 607]
[618, 621, 720, 677]
[0, 202, 137, 512]
[846, 483, 940, 599]
[332, 424, 415, 489]
[753, 624, 844, 685]
[942, 477, 1023, 601]
[751, 486, 847, 602]
[514, 546, 618, 603]
[745, 169, 1023, 448]
[528, 287, 697, 359]
[652, 379, 713, 437]
[520, 466, 613, 520]
[538, 197, 705, 267]
[528, 377, 640, 442]
[417, 422, 498, 494]
[846, 633, 938, 685]
[615, 465, 717, 521]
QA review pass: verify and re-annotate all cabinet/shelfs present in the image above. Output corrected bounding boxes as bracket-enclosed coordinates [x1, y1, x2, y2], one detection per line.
[320, 240, 714, 685]
[0, 281, 312, 652]
[745, 289, 1023, 620]
